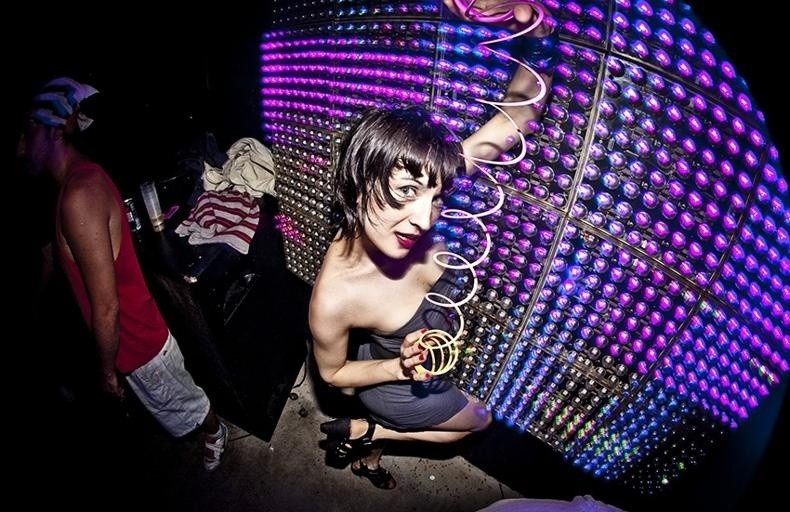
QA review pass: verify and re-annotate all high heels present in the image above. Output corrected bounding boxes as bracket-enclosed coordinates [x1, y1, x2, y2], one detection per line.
[352, 455, 397, 490]
[321, 416, 376, 468]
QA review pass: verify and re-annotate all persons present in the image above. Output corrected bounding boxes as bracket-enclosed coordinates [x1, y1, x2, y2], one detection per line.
[308, 0, 551, 489]
[16, 78, 228, 472]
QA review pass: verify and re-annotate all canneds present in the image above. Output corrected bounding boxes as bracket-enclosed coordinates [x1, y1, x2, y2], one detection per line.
[123, 197, 142, 233]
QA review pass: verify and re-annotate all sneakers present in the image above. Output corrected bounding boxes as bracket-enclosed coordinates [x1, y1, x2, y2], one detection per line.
[203, 423, 230, 473]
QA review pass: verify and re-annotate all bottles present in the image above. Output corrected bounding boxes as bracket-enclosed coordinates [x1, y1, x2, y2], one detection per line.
[140, 180, 164, 233]
